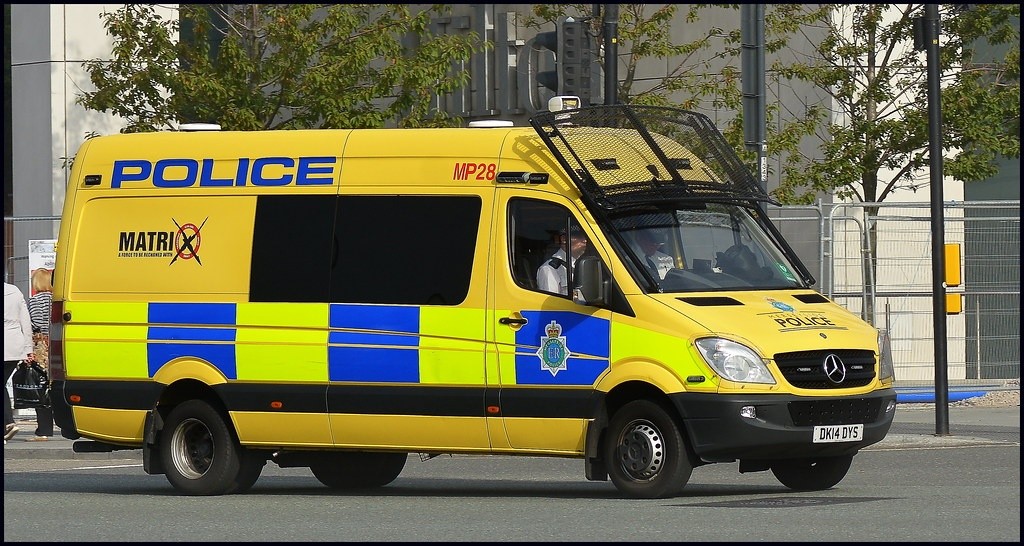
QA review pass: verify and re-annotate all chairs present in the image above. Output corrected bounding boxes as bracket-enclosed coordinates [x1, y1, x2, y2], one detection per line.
[519, 226, 549, 288]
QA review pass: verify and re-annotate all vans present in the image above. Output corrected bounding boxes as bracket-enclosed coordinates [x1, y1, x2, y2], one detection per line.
[49, 94, 896, 498]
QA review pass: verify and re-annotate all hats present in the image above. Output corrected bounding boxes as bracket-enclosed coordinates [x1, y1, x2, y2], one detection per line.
[639, 221, 670, 244]
[557, 223, 583, 236]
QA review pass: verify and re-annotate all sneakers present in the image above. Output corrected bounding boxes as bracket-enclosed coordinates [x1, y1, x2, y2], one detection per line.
[26, 435, 49, 441]
[4, 424, 20, 439]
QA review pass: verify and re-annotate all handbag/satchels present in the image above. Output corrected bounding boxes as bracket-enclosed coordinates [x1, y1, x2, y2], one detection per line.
[11, 361, 50, 409]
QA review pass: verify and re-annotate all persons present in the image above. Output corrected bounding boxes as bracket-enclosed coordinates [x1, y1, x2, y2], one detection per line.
[26, 267, 80, 440]
[3, 280, 35, 442]
[621, 208, 677, 286]
[536, 218, 588, 303]
